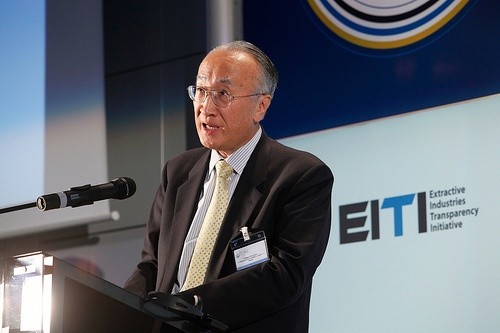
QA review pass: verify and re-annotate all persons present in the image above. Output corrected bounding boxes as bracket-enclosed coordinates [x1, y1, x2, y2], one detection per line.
[122, 42, 335, 333]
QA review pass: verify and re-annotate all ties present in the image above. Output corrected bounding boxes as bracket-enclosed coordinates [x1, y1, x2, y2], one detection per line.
[177, 160, 234, 294]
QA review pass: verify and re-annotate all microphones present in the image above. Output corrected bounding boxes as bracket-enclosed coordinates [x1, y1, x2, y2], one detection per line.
[36, 176, 137, 212]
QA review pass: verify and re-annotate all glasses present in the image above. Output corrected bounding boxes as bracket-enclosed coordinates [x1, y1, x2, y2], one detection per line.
[187, 85, 264, 107]
